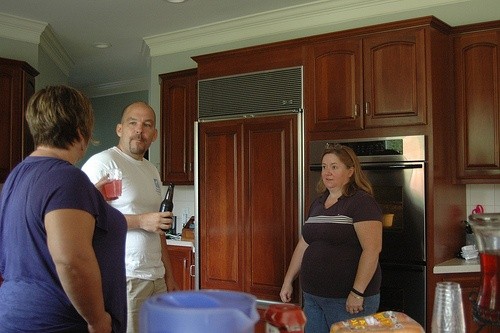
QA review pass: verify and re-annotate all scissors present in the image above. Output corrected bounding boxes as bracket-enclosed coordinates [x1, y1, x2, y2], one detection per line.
[472, 205, 483, 214]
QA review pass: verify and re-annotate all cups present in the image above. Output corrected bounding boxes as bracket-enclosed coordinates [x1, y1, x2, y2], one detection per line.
[100, 169, 122, 198]
[431, 281, 466, 333]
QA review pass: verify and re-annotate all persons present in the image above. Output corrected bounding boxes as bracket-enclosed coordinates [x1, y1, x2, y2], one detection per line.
[0, 85, 127, 333]
[280, 142, 382, 333]
[80, 100, 181, 333]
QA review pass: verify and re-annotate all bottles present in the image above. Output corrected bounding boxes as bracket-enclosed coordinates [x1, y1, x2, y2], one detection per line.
[468, 213, 500, 328]
[159, 183, 174, 232]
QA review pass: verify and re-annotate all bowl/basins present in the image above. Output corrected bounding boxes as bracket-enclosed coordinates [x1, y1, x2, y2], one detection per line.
[138, 290, 260, 333]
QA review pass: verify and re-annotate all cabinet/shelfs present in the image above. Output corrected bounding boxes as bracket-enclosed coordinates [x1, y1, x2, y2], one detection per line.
[164, 245, 195, 291]
[453, 20, 500, 184]
[441, 275, 500, 333]
[191, 14, 467, 333]
[159, 66, 195, 186]
[0, 56, 40, 185]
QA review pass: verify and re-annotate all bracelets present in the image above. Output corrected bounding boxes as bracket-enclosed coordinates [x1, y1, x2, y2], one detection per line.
[350, 292, 362, 299]
[351, 287, 364, 297]
